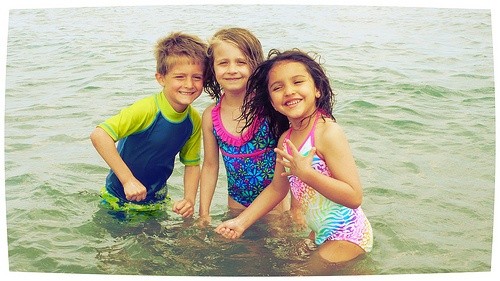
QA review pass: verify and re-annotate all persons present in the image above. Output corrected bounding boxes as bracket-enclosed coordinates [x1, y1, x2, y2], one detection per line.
[212, 47, 375, 276]
[193, 27, 305, 231]
[89, 29, 212, 229]
